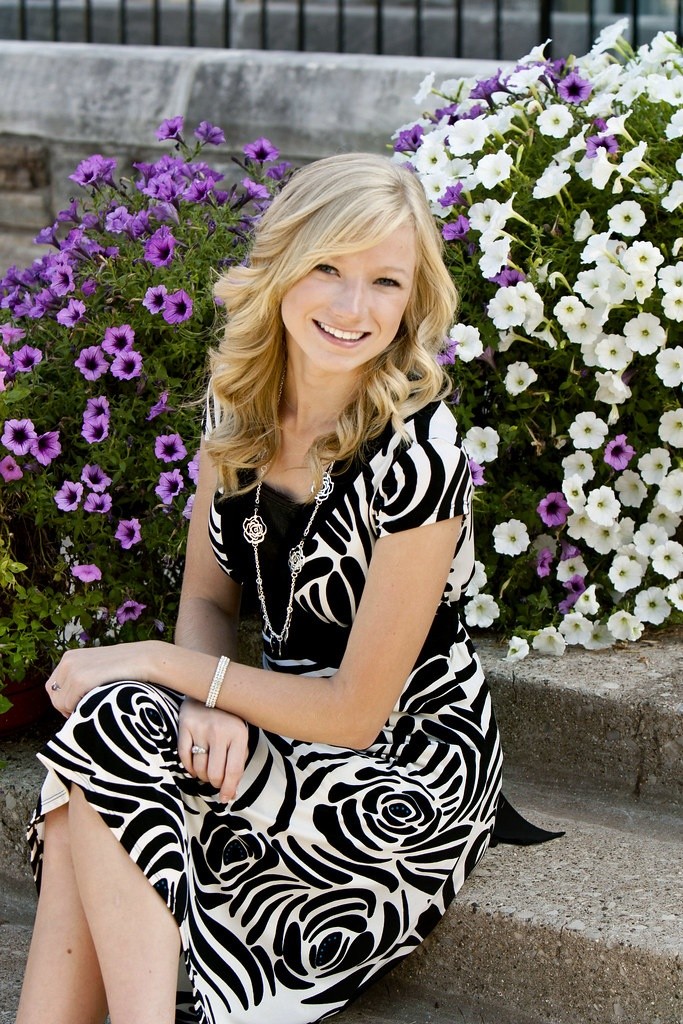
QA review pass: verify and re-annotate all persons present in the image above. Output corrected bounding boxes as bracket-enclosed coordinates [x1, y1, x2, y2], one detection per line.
[16, 153, 505, 1024]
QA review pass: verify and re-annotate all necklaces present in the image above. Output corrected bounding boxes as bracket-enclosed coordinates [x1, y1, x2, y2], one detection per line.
[243, 458, 336, 655]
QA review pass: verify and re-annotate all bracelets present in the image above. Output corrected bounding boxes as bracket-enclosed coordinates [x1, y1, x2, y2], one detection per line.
[206, 655, 231, 708]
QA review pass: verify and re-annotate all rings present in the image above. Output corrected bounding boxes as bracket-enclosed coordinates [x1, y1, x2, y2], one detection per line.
[192, 746, 208, 754]
[52, 681, 61, 691]
